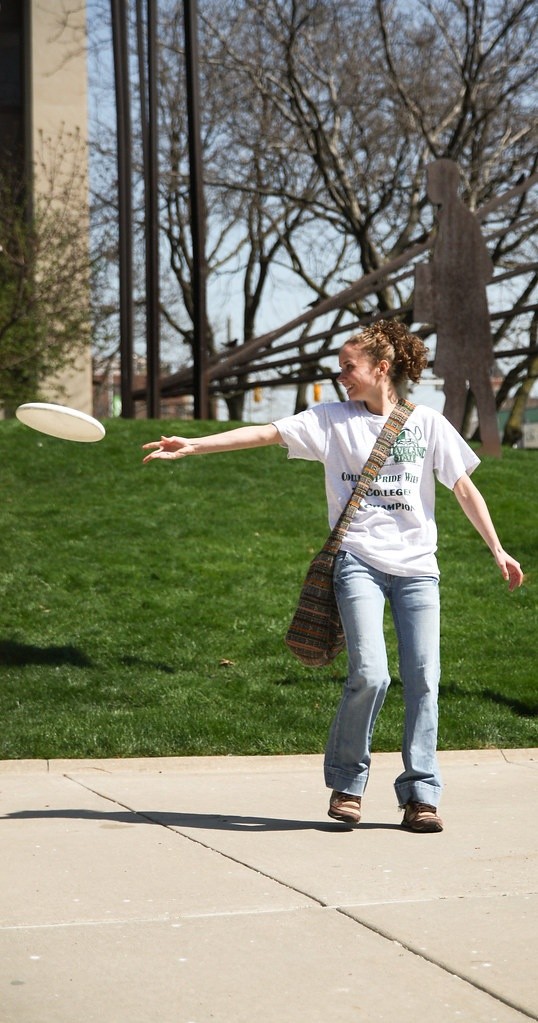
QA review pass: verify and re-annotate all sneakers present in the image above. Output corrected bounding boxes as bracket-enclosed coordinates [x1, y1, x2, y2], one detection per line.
[328, 789, 361, 825]
[401, 801, 443, 832]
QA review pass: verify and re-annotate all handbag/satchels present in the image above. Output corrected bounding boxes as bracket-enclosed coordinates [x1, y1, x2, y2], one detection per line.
[285, 549, 346, 667]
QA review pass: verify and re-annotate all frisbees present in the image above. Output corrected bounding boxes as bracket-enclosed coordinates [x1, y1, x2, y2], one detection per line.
[15, 402, 106, 443]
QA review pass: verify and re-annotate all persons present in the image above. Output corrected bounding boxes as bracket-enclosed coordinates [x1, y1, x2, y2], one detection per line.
[142, 319, 524, 832]
